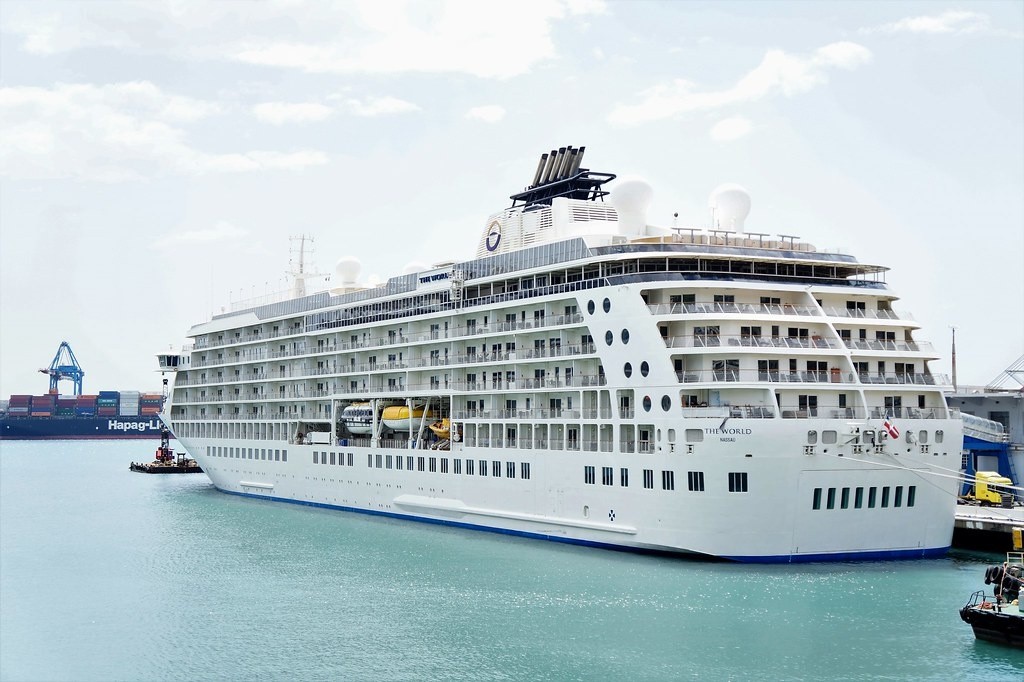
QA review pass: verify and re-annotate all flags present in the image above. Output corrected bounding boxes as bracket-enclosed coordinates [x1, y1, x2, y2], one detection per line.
[884, 416, 900, 438]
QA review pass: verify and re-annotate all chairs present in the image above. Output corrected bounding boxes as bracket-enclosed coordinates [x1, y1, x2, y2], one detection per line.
[906, 406, 919, 419]
[846, 408, 857, 419]
[783, 411, 796, 418]
[796, 411, 808, 419]
[876, 407, 885, 419]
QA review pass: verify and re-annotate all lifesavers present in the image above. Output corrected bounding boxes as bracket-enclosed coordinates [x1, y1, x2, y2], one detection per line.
[984, 565, 1017, 596]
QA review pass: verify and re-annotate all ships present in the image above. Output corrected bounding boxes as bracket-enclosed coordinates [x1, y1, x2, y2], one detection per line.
[157, 145, 963, 564]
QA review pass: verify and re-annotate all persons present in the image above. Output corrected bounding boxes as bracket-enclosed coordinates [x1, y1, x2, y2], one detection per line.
[298, 431, 304, 444]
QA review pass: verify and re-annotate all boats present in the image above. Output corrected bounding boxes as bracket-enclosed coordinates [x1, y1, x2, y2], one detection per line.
[960, 551, 1024, 647]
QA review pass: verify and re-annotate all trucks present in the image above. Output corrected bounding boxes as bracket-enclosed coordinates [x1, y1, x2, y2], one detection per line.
[975, 471, 1014, 507]
